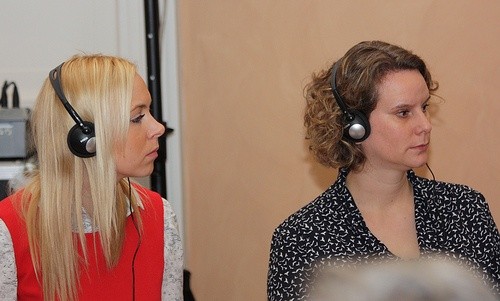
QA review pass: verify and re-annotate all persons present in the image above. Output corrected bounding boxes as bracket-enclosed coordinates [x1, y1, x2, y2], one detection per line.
[264, 39, 500, 301]
[1, 55, 186, 301]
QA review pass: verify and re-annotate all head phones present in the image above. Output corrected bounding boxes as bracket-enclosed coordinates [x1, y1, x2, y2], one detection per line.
[48, 62, 97, 158]
[328, 57, 371, 144]
